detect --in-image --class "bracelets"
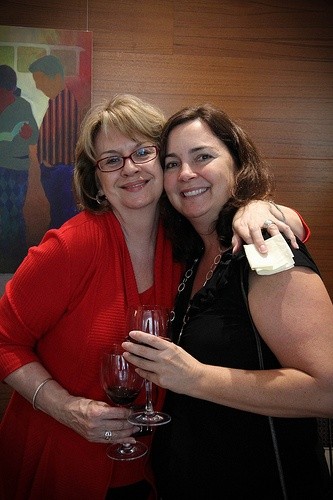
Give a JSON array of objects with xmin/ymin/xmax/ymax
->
[{"xmin": 33, "ymin": 378, "xmax": 56, "ymax": 414}]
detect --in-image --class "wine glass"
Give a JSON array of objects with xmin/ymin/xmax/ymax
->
[
  {"xmin": 127, "ymin": 305, "xmax": 171, "ymax": 426},
  {"xmin": 99, "ymin": 345, "xmax": 149, "ymax": 461}
]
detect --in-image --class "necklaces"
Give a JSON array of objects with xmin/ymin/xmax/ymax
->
[{"xmin": 169, "ymin": 241, "xmax": 233, "ymax": 347}]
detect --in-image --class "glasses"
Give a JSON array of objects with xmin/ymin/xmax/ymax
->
[{"xmin": 96, "ymin": 146, "xmax": 160, "ymax": 173}]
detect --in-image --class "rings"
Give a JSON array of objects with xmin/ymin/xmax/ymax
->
[
  {"xmin": 263, "ymin": 221, "xmax": 274, "ymax": 229},
  {"xmin": 105, "ymin": 430, "xmax": 112, "ymax": 441}
]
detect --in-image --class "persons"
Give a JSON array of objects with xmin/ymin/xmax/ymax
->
[
  {"xmin": 0, "ymin": 93, "xmax": 312, "ymax": 500},
  {"xmin": 118, "ymin": 106, "xmax": 332, "ymax": 500}
]
